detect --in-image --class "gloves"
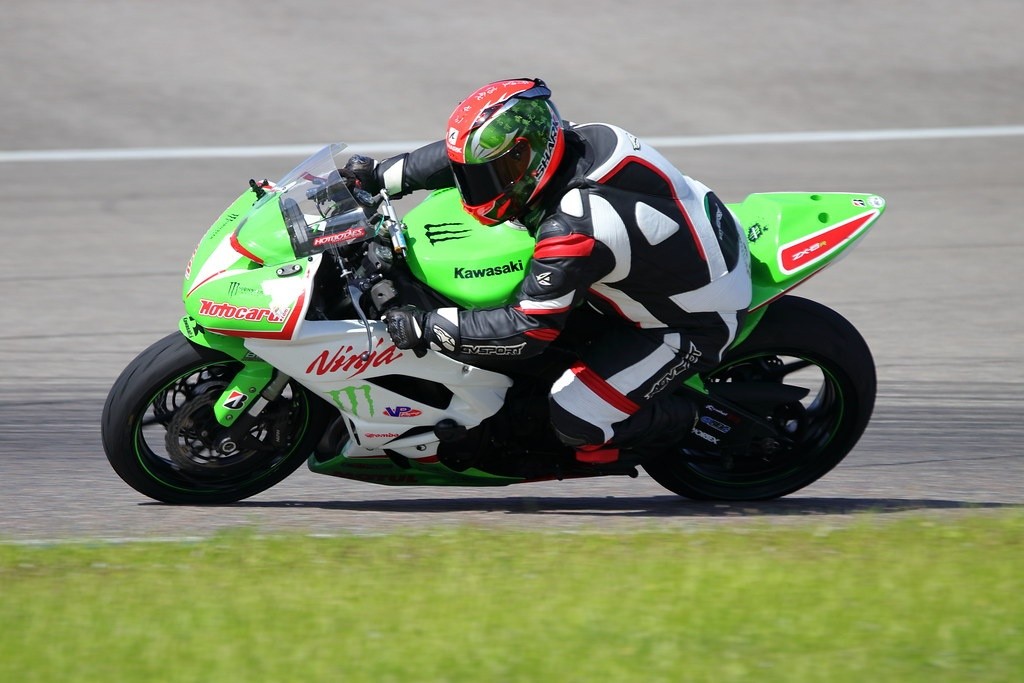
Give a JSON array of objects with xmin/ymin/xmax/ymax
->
[
  {"xmin": 381, "ymin": 304, "xmax": 462, "ymax": 355},
  {"xmin": 344, "ymin": 151, "xmax": 406, "ymax": 201}
]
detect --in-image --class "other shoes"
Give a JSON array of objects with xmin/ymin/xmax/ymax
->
[{"xmin": 637, "ymin": 395, "xmax": 701, "ymax": 451}]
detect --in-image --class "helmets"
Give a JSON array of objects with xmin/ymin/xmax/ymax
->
[{"xmin": 445, "ymin": 78, "xmax": 573, "ymax": 227}]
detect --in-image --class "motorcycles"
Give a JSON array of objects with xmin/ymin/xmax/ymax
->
[{"xmin": 99, "ymin": 142, "xmax": 886, "ymax": 507}]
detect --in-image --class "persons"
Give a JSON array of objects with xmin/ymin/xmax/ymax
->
[{"xmin": 346, "ymin": 78, "xmax": 753, "ymax": 475}]
